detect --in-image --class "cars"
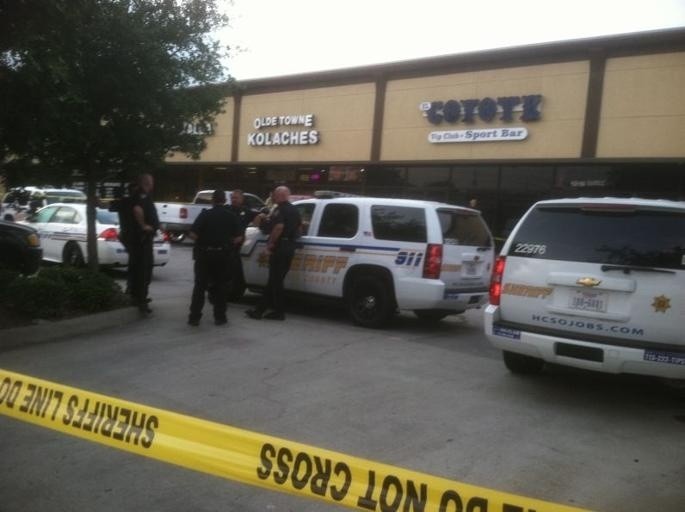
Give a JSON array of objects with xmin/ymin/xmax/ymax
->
[{"xmin": 483, "ymin": 197, "xmax": 685, "ymax": 380}]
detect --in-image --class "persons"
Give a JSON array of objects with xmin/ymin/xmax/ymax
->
[
  {"xmin": 94, "ymin": 175, "xmax": 161, "ymax": 313},
  {"xmin": 189, "ymin": 186, "xmax": 303, "ymax": 325}
]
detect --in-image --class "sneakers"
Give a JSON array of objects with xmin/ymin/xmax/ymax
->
[{"xmin": 244, "ymin": 308, "xmax": 285, "ymax": 319}]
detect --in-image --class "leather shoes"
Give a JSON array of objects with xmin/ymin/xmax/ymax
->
[
  {"xmin": 214, "ymin": 316, "xmax": 228, "ymax": 325},
  {"xmin": 187, "ymin": 320, "xmax": 200, "ymax": 326}
]
